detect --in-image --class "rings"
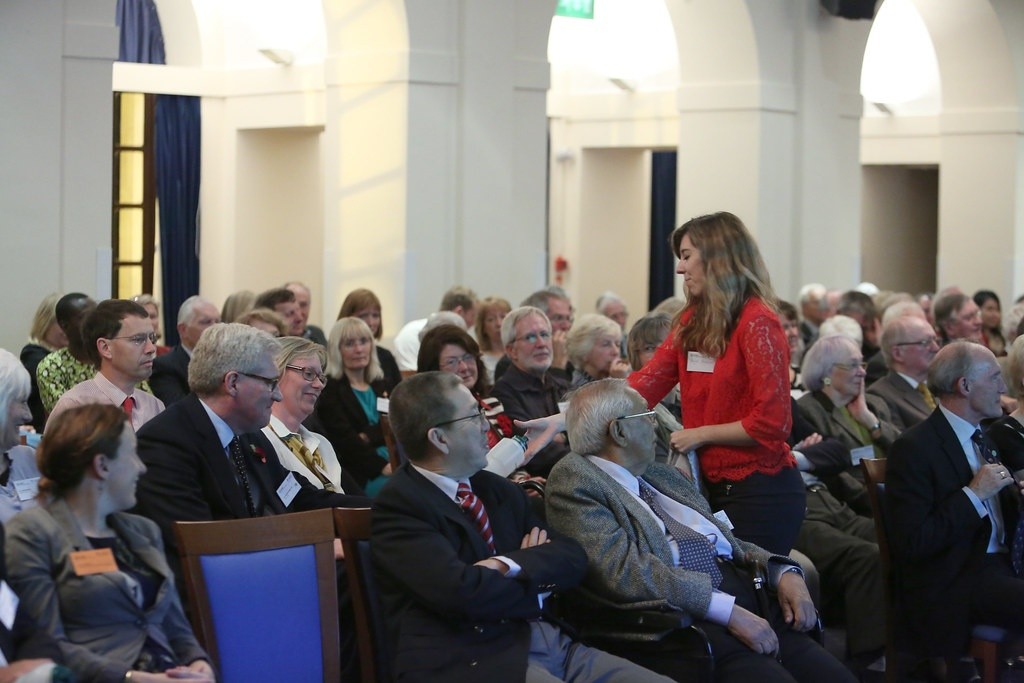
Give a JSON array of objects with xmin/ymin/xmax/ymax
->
[{"xmin": 999, "ymin": 472, "xmax": 1007, "ymax": 479}]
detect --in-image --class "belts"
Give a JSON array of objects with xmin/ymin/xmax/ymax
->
[
  {"xmin": 714, "ymin": 555, "xmax": 729, "ymax": 567},
  {"xmin": 806, "ymin": 485, "xmax": 825, "ymax": 495}
]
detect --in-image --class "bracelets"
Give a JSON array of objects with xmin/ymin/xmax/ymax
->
[
  {"xmin": 124, "ymin": 670, "xmax": 133, "ymax": 682},
  {"xmin": 788, "ymin": 568, "xmax": 799, "ymax": 572},
  {"xmin": 867, "ymin": 424, "xmax": 879, "ymax": 432}
]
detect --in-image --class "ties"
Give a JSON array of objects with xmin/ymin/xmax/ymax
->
[
  {"xmin": 228, "ymin": 435, "xmax": 258, "ymax": 519},
  {"xmin": 970, "ymin": 429, "xmax": 1024, "ymax": 576},
  {"xmin": 918, "ymin": 382, "xmax": 936, "ymax": 412},
  {"xmin": 639, "ymin": 477, "xmax": 723, "ymax": 589},
  {"xmin": 457, "ymin": 482, "xmax": 496, "ymax": 558},
  {"xmin": 122, "ymin": 396, "xmax": 134, "ymax": 433}
]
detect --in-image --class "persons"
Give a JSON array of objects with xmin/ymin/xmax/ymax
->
[
  {"xmin": 513, "ymin": 212, "xmax": 807, "ymax": 554},
  {"xmin": 0, "ymin": 282, "xmax": 1024, "ymax": 683}
]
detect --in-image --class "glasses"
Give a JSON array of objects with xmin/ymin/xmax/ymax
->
[
  {"xmin": 239, "ymin": 371, "xmax": 279, "ymax": 393},
  {"xmin": 440, "ymin": 352, "xmax": 475, "ymax": 371},
  {"xmin": 835, "ymin": 362, "xmax": 869, "ymax": 372},
  {"xmin": 432, "ymin": 407, "xmax": 486, "ymax": 429},
  {"xmin": 286, "ymin": 363, "xmax": 328, "ymax": 389},
  {"xmin": 893, "ymin": 336, "xmax": 944, "ymax": 351},
  {"xmin": 108, "ymin": 332, "xmax": 158, "ymax": 347},
  {"xmin": 511, "ymin": 330, "xmax": 551, "ymax": 343},
  {"xmin": 615, "ymin": 408, "xmax": 656, "ymax": 425},
  {"xmin": 549, "ymin": 313, "xmax": 575, "ymax": 324}
]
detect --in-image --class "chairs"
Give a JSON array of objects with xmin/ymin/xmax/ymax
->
[
  {"xmin": 334, "ymin": 507, "xmax": 381, "ymax": 683},
  {"xmin": 172, "ymin": 507, "xmax": 341, "ymax": 683},
  {"xmin": 860, "ymin": 457, "xmax": 1011, "ymax": 683}
]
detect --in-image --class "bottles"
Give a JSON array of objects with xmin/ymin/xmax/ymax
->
[{"xmin": 481, "ymin": 435, "xmax": 528, "ymax": 478}]
